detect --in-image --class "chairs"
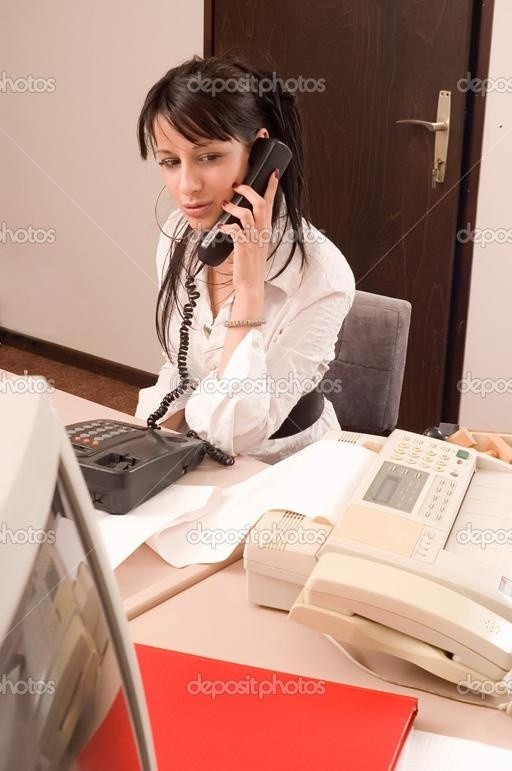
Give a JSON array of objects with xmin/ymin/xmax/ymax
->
[{"xmin": 314, "ymin": 239, "xmax": 414, "ymax": 438}]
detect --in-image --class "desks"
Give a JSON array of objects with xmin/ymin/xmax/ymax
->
[{"xmin": 2, "ymin": 368, "xmax": 512, "ymax": 771}]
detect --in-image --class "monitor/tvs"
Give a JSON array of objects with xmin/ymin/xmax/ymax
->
[{"xmin": 0, "ymin": 365, "xmax": 158, "ymax": 771}]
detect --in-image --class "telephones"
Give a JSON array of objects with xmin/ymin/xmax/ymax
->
[
  {"xmin": 197, "ymin": 138, "xmax": 293, "ymax": 266},
  {"xmin": 243, "ymin": 428, "xmax": 512, "ymax": 697}
]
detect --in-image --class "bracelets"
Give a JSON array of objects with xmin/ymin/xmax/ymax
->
[{"xmin": 222, "ymin": 318, "xmax": 270, "ymax": 329}]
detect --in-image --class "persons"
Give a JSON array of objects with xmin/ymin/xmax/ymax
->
[{"xmin": 133, "ymin": 48, "xmax": 356, "ymax": 467}]
